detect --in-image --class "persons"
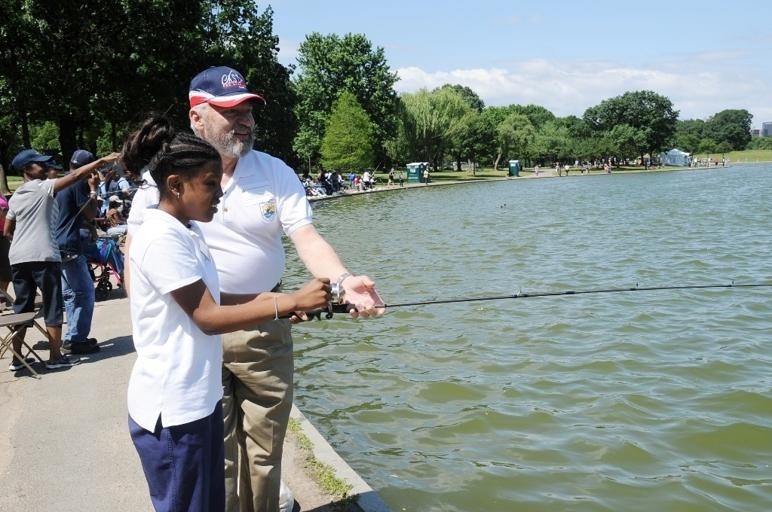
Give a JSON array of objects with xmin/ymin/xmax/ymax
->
[
  {"xmin": 552, "ymin": 157, "xmax": 612, "ymax": 176},
  {"xmin": 423, "ymin": 168, "xmax": 429, "ymax": 184},
  {"xmin": 118, "ymin": 114, "xmax": 333, "ymax": 511},
  {"xmin": 0, "ymin": 147, "xmax": 143, "ymax": 372},
  {"xmin": 123, "ymin": 63, "xmax": 386, "ymax": 511},
  {"xmin": 682, "ymin": 155, "xmax": 717, "ymax": 169},
  {"xmin": 304, "ymin": 167, "xmax": 404, "ymax": 197}
]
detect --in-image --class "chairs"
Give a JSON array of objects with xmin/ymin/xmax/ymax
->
[{"xmin": 0, "ymin": 287, "xmax": 49, "ymax": 380}]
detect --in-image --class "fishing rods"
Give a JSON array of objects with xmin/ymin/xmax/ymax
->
[
  {"xmin": 280, "ymin": 282, "xmax": 770, "ymax": 320},
  {"xmin": 68, "ymin": 96, "xmax": 183, "ymax": 225},
  {"xmin": 372, "ymin": 159, "xmax": 386, "ymax": 176}
]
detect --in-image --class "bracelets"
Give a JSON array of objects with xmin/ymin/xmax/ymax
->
[
  {"xmin": 274, "ymin": 295, "xmax": 280, "ymax": 321},
  {"xmin": 331, "ymin": 272, "xmax": 352, "ymax": 294}
]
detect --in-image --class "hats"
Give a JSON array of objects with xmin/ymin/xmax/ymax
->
[
  {"xmin": 109, "ymin": 195, "xmax": 123, "ymax": 204},
  {"xmin": 187, "ymin": 65, "xmax": 266, "ymax": 114},
  {"xmin": 97, "ymin": 196, "xmax": 105, "ymax": 202},
  {"xmin": 11, "ymin": 149, "xmax": 54, "ymax": 170},
  {"xmin": 71, "ymin": 150, "xmax": 93, "ymax": 166}
]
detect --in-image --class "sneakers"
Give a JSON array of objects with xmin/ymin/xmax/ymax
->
[
  {"xmin": 45, "ymin": 337, "xmax": 100, "ymax": 369},
  {"xmin": 8, "ymin": 358, "xmax": 35, "ymax": 370}
]
